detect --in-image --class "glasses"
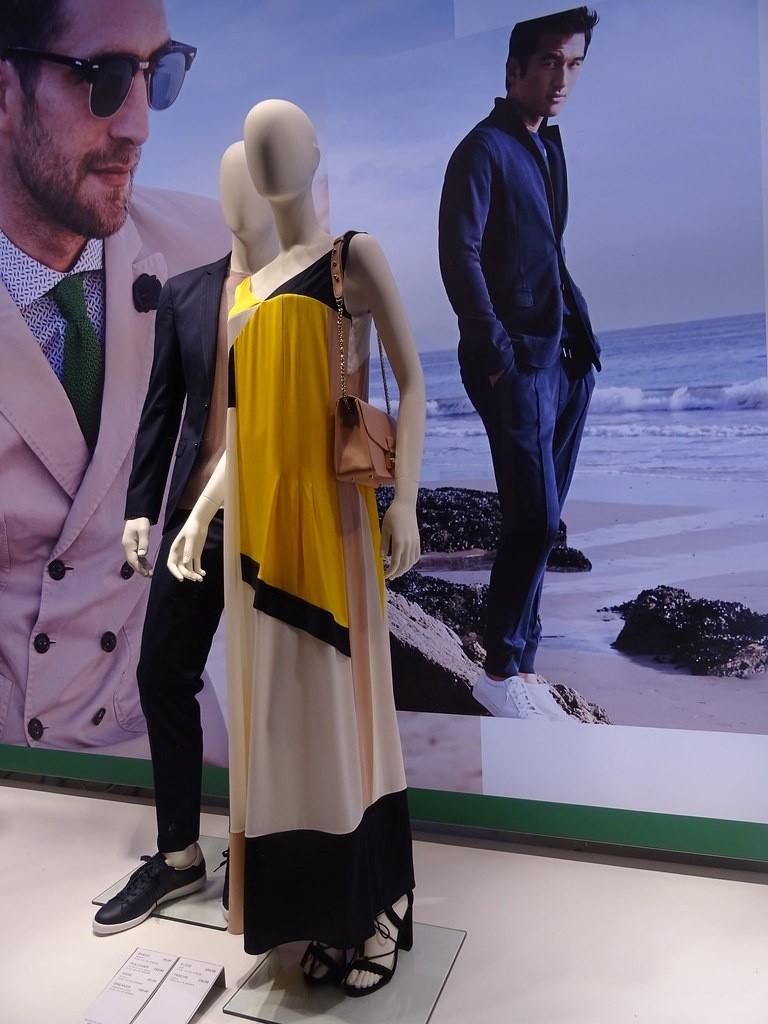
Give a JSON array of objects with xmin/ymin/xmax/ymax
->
[{"xmin": 0, "ymin": 40, "xmax": 197, "ymax": 119}]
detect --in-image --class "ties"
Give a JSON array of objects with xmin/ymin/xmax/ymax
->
[{"xmin": 44, "ymin": 272, "xmax": 105, "ymax": 458}]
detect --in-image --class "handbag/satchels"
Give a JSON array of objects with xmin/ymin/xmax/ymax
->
[{"xmin": 334, "ymin": 395, "xmax": 398, "ymax": 487}]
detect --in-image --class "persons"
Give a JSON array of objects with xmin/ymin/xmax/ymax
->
[
  {"xmin": 89, "ymin": 97, "xmax": 427, "ymax": 998},
  {"xmin": 435, "ymin": 4, "xmax": 604, "ymax": 723},
  {"xmin": 0, "ymin": 1, "xmax": 233, "ymax": 762}
]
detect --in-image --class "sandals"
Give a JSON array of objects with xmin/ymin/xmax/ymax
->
[
  {"xmin": 341, "ymin": 889, "xmax": 414, "ymax": 997},
  {"xmin": 300, "ymin": 939, "xmax": 364, "ymax": 985}
]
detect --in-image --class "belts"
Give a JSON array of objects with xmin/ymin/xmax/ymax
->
[{"xmin": 558, "ymin": 347, "xmax": 581, "ymax": 359}]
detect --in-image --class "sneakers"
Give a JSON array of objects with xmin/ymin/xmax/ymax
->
[
  {"xmin": 214, "ymin": 846, "xmax": 230, "ymax": 921},
  {"xmin": 472, "ymin": 669, "xmax": 578, "ymax": 722},
  {"xmin": 93, "ymin": 842, "xmax": 207, "ymax": 934}
]
